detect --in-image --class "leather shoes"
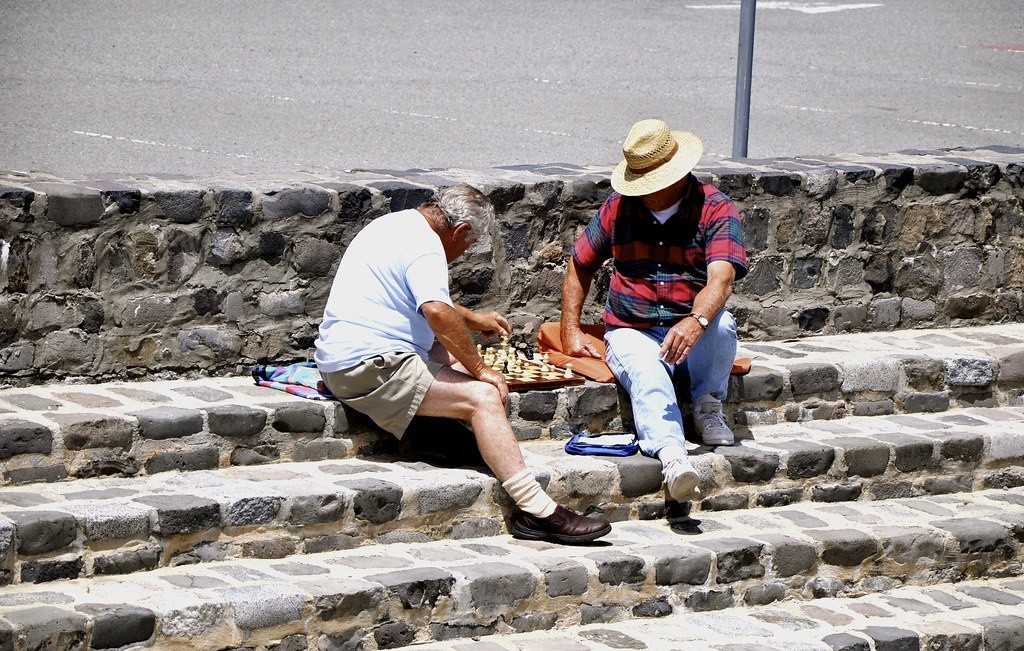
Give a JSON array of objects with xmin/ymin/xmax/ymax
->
[
  {"xmin": 441, "ymin": 418, "xmax": 480, "ymax": 460},
  {"xmin": 512, "ymin": 505, "xmax": 612, "ymax": 542}
]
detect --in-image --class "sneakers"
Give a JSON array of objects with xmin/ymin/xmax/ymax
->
[
  {"xmin": 661, "ymin": 457, "xmax": 701, "ymax": 504},
  {"xmin": 693, "ymin": 403, "xmax": 734, "ymax": 445}
]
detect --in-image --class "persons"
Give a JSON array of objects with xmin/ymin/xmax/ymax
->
[
  {"xmin": 315, "ymin": 184, "xmax": 613, "ymax": 543},
  {"xmin": 561, "ymin": 118, "xmax": 749, "ymax": 503}
]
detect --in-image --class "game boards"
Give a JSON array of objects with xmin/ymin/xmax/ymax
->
[{"xmin": 462, "ymin": 345, "xmax": 588, "ymax": 393}]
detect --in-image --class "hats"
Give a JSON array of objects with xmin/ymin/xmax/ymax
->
[{"xmin": 611, "ymin": 119, "xmax": 702, "ymax": 197}]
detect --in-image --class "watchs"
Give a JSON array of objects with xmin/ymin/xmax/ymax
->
[{"xmin": 688, "ymin": 313, "xmax": 709, "ymax": 331}]
